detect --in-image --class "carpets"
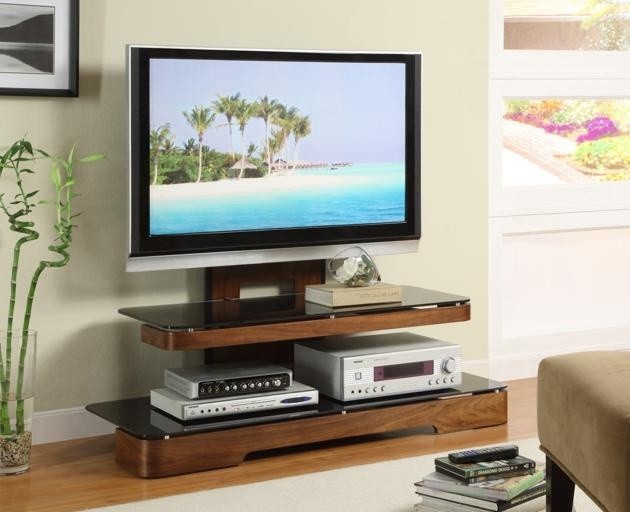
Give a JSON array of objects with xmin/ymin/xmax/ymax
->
[{"xmin": 78, "ymin": 437, "xmax": 603, "ymax": 512}]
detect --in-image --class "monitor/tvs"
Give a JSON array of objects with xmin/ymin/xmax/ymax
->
[{"xmin": 126, "ymin": 43, "xmax": 423, "ymax": 273}]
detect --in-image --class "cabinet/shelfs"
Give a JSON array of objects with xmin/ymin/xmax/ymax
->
[{"xmin": 86, "ymin": 281, "xmax": 507, "ymax": 480}]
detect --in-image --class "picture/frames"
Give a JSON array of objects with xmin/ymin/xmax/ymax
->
[{"xmin": 0, "ymin": 0, "xmax": 79, "ymax": 97}]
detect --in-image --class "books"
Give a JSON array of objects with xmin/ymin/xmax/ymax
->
[
  {"xmin": 305, "ymin": 281, "xmax": 401, "ymax": 308},
  {"xmin": 413, "ymin": 454, "xmax": 547, "ymax": 512}
]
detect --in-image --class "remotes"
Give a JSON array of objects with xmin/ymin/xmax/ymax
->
[{"xmin": 453, "ymin": 445, "xmax": 518, "ymax": 464}]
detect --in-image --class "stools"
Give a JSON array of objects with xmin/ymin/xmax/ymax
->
[{"xmin": 536, "ymin": 349, "xmax": 630, "ymax": 512}]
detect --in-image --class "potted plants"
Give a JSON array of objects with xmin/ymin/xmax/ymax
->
[{"xmin": 0, "ymin": 134, "xmax": 107, "ymax": 477}]
